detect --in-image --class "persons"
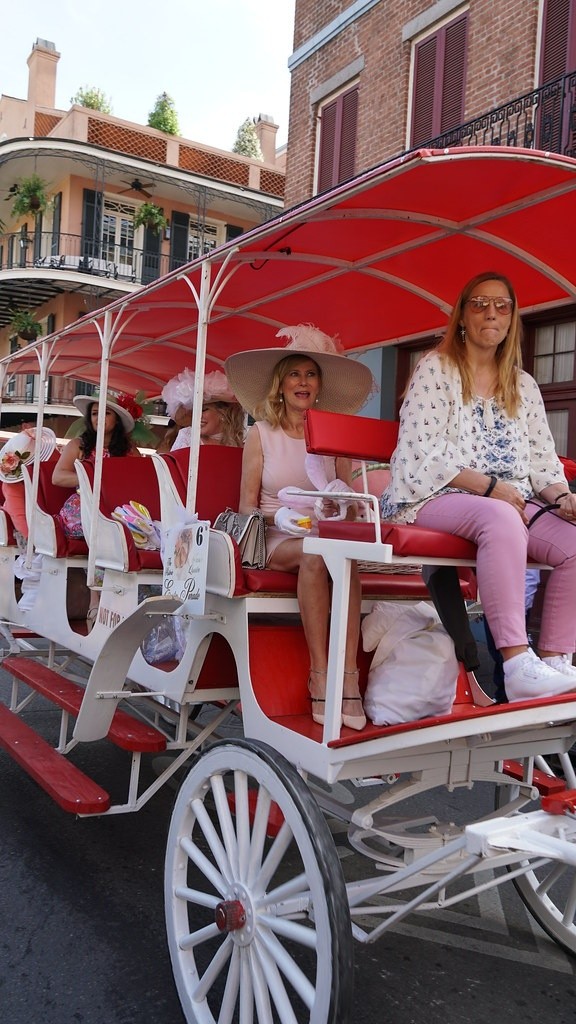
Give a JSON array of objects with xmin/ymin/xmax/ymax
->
[
  {"xmin": 51, "ymin": 393, "xmax": 141, "ymax": 631},
  {"xmin": 162, "ymin": 369, "xmax": 245, "ymax": 452},
  {"xmin": 382, "ymin": 271, "xmax": 576, "ymax": 703},
  {"xmin": 0, "ymin": 427, "xmax": 56, "ymax": 549},
  {"xmin": 225, "ymin": 326, "xmax": 373, "ymax": 730}
]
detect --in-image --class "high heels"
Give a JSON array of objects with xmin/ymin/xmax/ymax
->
[
  {"xmin": 86, "ymin": 607, "xmax": 98, "ymax": 634},
  {"xmin": 341, "ymin": 669, "xmax": 367, "ymax": 730},
  {"xmin": 307, "ymin": 669, "xmax": 327, "ymax": 725}
]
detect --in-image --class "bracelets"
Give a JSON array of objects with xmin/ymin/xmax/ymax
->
[
  {"xmin": 554, "ymin": 493, "xmax": 568, "ymax": 503},
  {"xmin": 483, "ymin": 476, "xmax": 497, "ymax": 496}
]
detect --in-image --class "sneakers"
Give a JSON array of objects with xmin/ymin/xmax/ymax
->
[
  {"xmin": 502, "ymin": 647, "xmax": 576, "ymax": 704},
  {"xmin": 540, "ymin": 654, "xmax": 576, "ymax": 677}
]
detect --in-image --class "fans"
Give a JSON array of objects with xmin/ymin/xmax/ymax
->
[{"xmin": 117, "ymin": 178, "xmax": 156, "ymax": 198}]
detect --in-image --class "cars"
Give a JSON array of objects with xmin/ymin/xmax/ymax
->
[{"xmin": 0, "ymin": 403, "xmax": 171, "ymax": 456}]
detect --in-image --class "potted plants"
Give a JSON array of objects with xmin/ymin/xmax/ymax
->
[
  {"xmin": 11, "ymin": 176, "xmax": 55, "ymax": 223},
  {"xmin": 7, "ymin": 307, "xmax": 43, "ymax": 341},
  {"xmin": 132, "ymin": 203, "xmax": 166, "ymax": 233}
]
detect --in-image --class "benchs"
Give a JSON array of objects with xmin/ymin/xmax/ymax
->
[
  {"xmin": 72, "ymin": 458, "xmax": 164, "ymax": 636},
  {"xmin": 303, "ymin": 408, "xmax": 576, "ymax": 743},
  {"xmin": 150, "ymin": 444, "xmax": 333, "ymax": 719},
  {"xmin": 1, "ymin": 436, "xmax": 91, "ymax": 625}
]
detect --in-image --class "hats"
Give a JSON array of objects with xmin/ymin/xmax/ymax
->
[
  {"xmin": 160, "ymin": 367, "xmax": 239, "ymax": 427},
  {"xmin": 0, "ymin": 427, "xmax": 56, "ymax": 484},
  {"xmin": 223, "ymin": 322, "xmax": 379, "ymax": 424},
  {"xmin": 64, "ymin": 390, "xmax": 160, "ymax": 446}
]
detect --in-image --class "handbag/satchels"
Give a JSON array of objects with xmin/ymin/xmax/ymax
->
[
  {"xmin": 60, "ymin": 493, "xmax": 84, "ymax": 539},
  {"xmin": 212, "ymin": 507, "xmax": 265, "ymax": 570}
]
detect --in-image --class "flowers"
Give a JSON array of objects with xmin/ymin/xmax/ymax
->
[
  {"xmin": 115, "ymin": 391, "xmax": 157, "ymax": 444},
  {"xmin": 0, "ymin": 450, "xmax": 31, "ymax": 478}
]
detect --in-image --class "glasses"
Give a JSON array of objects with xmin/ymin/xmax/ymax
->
[{"xmin": 463, "ymin": 296, "xmax": 514, "ymax": 315}]
